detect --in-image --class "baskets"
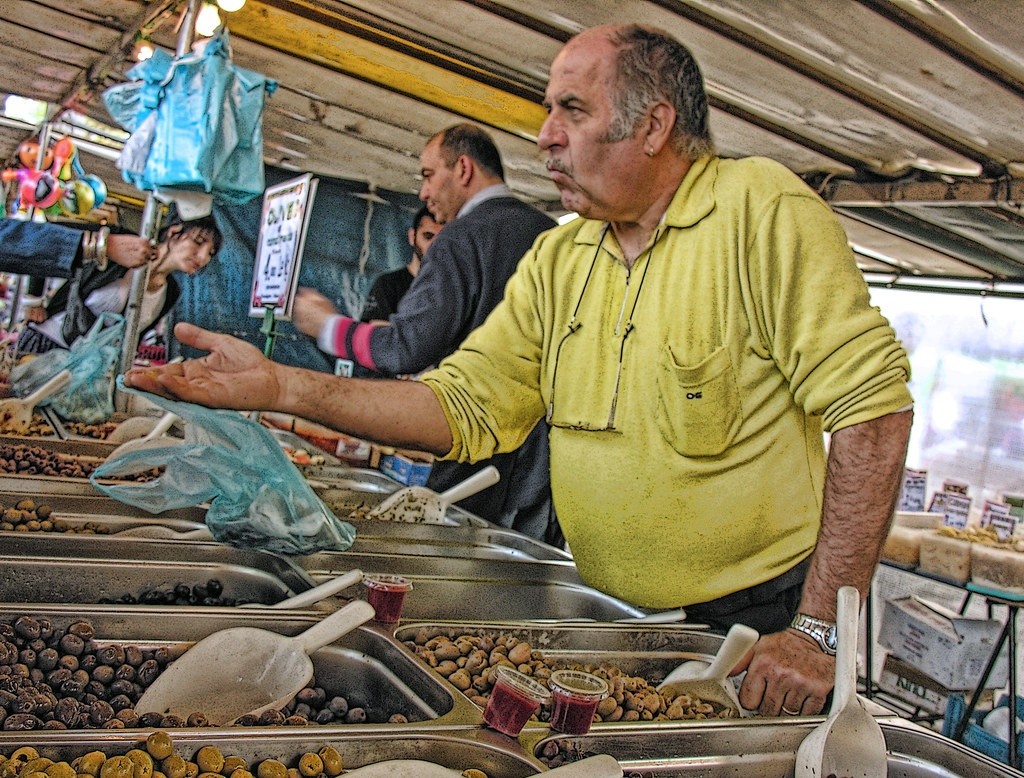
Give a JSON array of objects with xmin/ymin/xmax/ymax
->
[{"xmin": 942, "ymin": 694, "xmax": 1024, "ymax": 769}]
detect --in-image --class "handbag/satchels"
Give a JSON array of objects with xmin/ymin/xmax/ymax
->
[
  {"xmin": 89, "ymin": 372, "xmax": 358, "ymax": 556},
  {"xmin": 63, "ymin": 267, "xmax": 107, "ymax": 344},
  {"xmin": 11, "ymin": 311, "xmax": 126, "ymax": 424}
]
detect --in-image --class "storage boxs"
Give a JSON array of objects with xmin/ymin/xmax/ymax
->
[
  {"xmin": 964, "ymin": 709, "xmax": 1024, "ymax": 774},
  {"xmin": 881, "ymin": 653, "xmax": 993, "ymax": 715},
  {"xmin": 878, "ymin": 594, "xmax": 1010, "ymax": 692},
  {"xmin": 882, "ymin": 521, "xmax": 1024, "ymax": 601},
  {"xmin": 260, "ymin": 413, "xmax": 435, "ymax": 486}
]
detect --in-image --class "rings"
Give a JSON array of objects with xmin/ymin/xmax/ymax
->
[{"xmin": 782, "ymin": 706, "xmax": 799, "ymax": 716}]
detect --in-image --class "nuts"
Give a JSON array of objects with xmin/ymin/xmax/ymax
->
[{"xmin": 0, "ymin": 419, "xmax": 738, "ymax": 778}]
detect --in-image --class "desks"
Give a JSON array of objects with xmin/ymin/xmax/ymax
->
[{"xmin": 866, "ymin": 556, "xmax": 1024, "ymax": 768}]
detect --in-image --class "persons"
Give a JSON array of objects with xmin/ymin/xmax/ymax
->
[
  {"xmin": 122, "ymin": 21, "xmax": 915, "ymax": 722},
  {"xmin": 16, "ymin": 210, "xmax": 223, "ymax": 360},
  {"xmin": 292, "ymin": 124, "xmax": 559, "ymax": 551},
  {"xmin": 348, "ymin": 206, "xmax": 448, "ymax": 379},
  {"xmin": 0, "ymin": 218, "xmax": 158, "ymax": 279}
]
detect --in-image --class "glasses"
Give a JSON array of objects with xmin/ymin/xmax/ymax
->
[{"xmin": 544, "ymin": 325, "xmax": 637, "ymax": 434}]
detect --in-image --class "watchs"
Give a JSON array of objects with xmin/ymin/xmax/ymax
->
[{"xmin": 791, "ymin": 613, "xmax": 838, "ymax": 656}]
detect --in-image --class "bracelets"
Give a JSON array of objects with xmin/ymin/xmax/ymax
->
[{"xmin": 83, "ymin": 226, "xmax": 110, "ymax": 271}]
